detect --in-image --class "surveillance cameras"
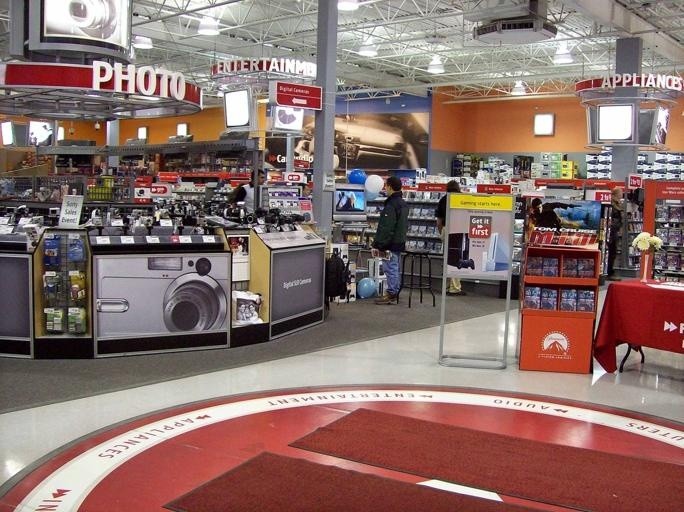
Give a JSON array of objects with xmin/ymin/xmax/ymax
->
[
  {"xmin": 68, "ymin": 127, "xmax": 74, "ymax": 135},
  {"xmin": 94, "ymin": 122, "xmax": 101, "ymax": 131}
]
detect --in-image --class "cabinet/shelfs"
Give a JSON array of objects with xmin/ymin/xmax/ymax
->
[
  {"xmin": 515, "ymin": 198, "xmax": 602, "ymax": 374},
  {"xmin": 0, "ymin": 137, "xmax": 684, "ymax": 310}
]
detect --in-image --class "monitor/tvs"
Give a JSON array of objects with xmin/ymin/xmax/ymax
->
[
  {"xmin": 1, "ymin": 121, "xmax": 15, "ymax": 146},
  {"xmin": 176, "ymin": 123, "xmax": 187, "ymax": 136},
  {"xmin": 137, "ymin": 127, "xmax": 147, "ymax": 140},
  {"xmin": 272, "ymin": 106, "xmax": 304, "ymax": 131},
  {"xmin": 27, "ymin": 0, "xmax": 134, "ymax": 64},
  {"xmin": 223, "ymin": 89, "xmax": 255, "ymax": 132},
  {"xmin": 332, "ymin": 189, "xmax": 367, "ymax": 214},
  {"xmin": 585, "ymin": 102, "xmax": 672, "ymax": 144},
  {"xmin": 25, "ymin": 120, "xmax": 57, "ymax": 148},
  {"xmin": 532, "ymin": 113, "xmax": 554, "ymax": 135}
]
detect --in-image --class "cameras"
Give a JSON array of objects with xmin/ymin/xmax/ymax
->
[
  {"xmin": 95, "ymin": 257, "xmax": 231, "ymax": 342},
  {"xmin": 44, "ymin": 0, "xmax": 129, "ymax": 48}
]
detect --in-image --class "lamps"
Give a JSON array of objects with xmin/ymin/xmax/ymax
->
[
  {"xmin": 197, "ymin": 3, "xmax": 220, "ymax": 37},
  {"xmin": 286, "ymin": 58, "xmax": 318, "ymax": 78},
  {"xmin": 338, "ymin": 2, "xmax": 363, "ymax": 12},
  {"xmin": 553, "ymin": 41, "xmax": 574, "ymax": 65},
  {"xmin": 133, "ymin": 35, "xmax": 154, "ymax": 50},
  {"xmin": 427, "ymin": 54, "xmax": 445, "ymax": 75},
  {"xmin": 511, "ymin": 81, "xmax": 527, "ymax": 97},
  {"xmin": 358, "ymin": 35, "xmax": 378, "ymax": 58}
]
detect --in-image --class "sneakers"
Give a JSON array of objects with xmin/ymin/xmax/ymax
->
[
  {"xmin": 446, "ymin": 290, "xmax": 468, "ymax": 296},
  {"xmin": 375, "ymin": 291, "xmax": 392, "ymax": 298},
  {"xmin": 375, "ymin": 295, "xmax": 398, "ymax": 305}
]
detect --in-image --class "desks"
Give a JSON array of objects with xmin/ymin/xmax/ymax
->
[{"xmin": 591, "ymin": 276, "xmax": 684, "ymax": 372}]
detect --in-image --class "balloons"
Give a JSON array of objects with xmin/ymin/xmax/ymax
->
[
  {"xmin": 357, "ymin": 277, "xmax": 375, "ymax": 299},
  {"xmin": 347, "ymin": 169, "xmax": 384, "ymax": 200}
]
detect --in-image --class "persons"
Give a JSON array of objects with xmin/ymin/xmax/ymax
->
[
  {"xmin": 529, "ymin": 198, "xmax": 582, "ymax": 230},
  {"xmin": 435, "ymin": 181, "xmax": 466, "ymax": 295},
  {"xmin": 340, "ymin": 192, "xmax": 355, "ymax": 210},
  {"xmin": 605, "ymin": 188, "xmax": 624, "ymax": 281},
  {"xmin": 371, "ymin": 177, "xmax": 409, "ymax": 305},
  {"xmin": 237, "ymin": 303, "xmax": 258, "ymax": 321},
  {"xmin": 235, "ymin": 170, "xmax": 265, "ymax": 213}
]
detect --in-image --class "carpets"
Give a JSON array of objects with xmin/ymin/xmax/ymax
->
[
  {"xmin": 159, "ymin": 448, "xmax": 550, "ymax": 512},
  {"xmin": 285, "ymin": 406, "xmax": 684, "ymax": 511}
]
